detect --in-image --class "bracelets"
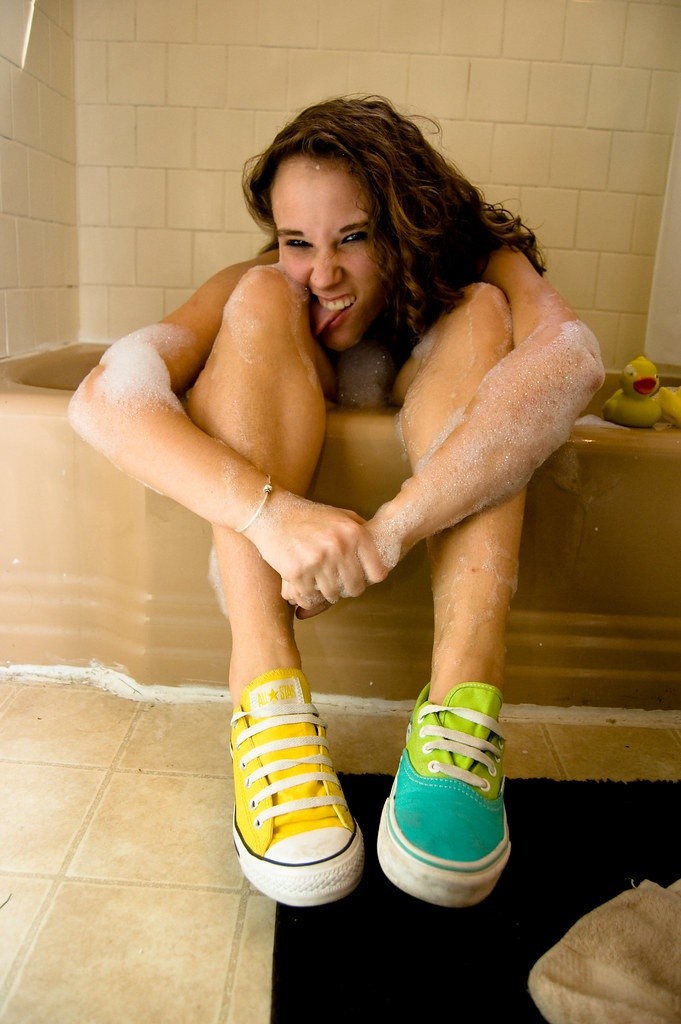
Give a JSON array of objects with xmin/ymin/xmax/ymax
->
[{"xmin": 237, "ymin": 476, "xmax": 273, "ymax": 537}]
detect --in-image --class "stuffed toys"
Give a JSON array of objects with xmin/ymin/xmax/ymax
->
[{"xmin": 600, "ymin": 356, "xmax": 681, "ymax": 432}]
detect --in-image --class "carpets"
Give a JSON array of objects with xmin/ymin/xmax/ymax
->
[{"xmin": 269, "ymin": 776, "xmax": 681, "ymax": 1024}]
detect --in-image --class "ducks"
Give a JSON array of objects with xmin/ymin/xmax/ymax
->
[{"xmin": 603, "ymin": 356, "xmax": 662, "ymax": 430}]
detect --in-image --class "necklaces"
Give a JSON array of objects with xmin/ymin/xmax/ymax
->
[{"xmin": 336, "ymin": 356, "xmax": 387, "ymax": 408}]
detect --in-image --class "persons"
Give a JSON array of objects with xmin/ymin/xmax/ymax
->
[{"xmin": 67, "ymin": 95, "xmax": 604, "ymax": 907}]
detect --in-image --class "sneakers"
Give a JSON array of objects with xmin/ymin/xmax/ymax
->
[{"xmin": 230, "ymin": 670, "xmax": 513, "ymax": 908}]
